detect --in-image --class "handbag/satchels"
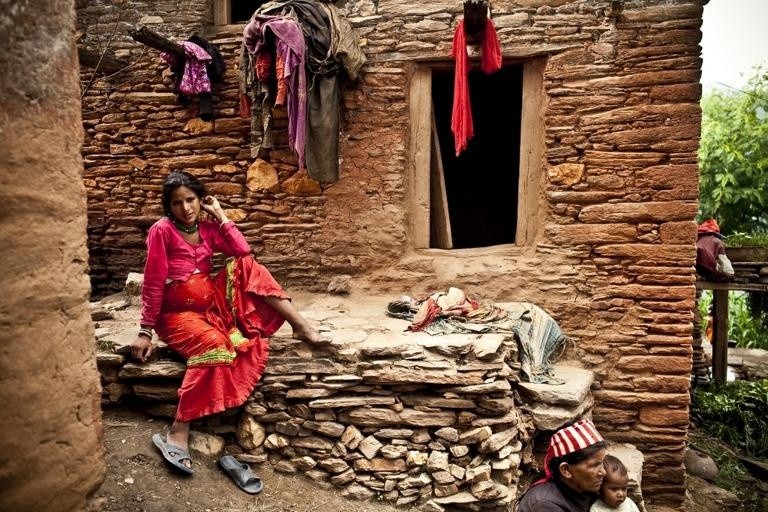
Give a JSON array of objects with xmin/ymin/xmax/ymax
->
[{"xmin": 715, "ymin": 254, "xmax": 735, "ymax": 278}]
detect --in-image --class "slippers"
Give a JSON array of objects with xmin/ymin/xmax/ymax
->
[
  {"xmin": 152, "ymin": 434, "xmax": 194, "ymax": 474},
  {"xmin": 219, "ymin": 455, "xmax": 264, "ymax": 494}
]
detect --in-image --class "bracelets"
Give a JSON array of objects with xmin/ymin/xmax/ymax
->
[
  {"xmin": 220, "ymin": 220, "xmax": 232, "ymax": 228},
  {"xmin": 138, "ymin": 329, "xmax": 153, "ymax": 341}
]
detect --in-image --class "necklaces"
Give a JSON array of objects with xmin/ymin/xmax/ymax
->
[{"xmin": 172, "ymin": 219, "xmax": 198, "ymax": 233}]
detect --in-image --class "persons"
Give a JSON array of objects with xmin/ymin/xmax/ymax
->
[
  {"xmin": 516, "ymin": 416, "xmax": 608, "ymax": 512},
  {"xmin": 132, "ymin": 172, "xmax": 332, "ymax": 469},
  {"xmin": 589, "ymin": 455, "xmax": 640, "ymax": 512}
]
